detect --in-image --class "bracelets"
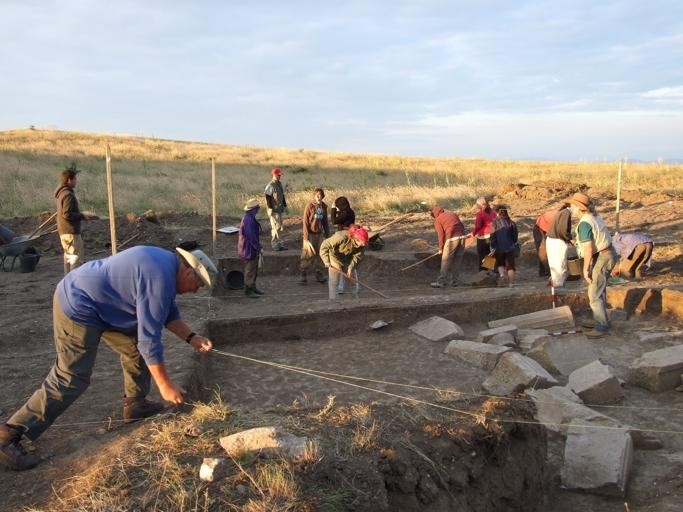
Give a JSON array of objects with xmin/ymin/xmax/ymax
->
[{"xmin": 185, "ymin": 332, "xmax": 195, "ymax": 344}]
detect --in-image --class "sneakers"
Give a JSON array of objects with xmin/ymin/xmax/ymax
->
[
  {"xmin": 584, "ymin": 331, "xmax": 609, "ymax": 339},
  {"xmin": 0, "ymin": 424, "xmax": 40, "ymax": 469},
  {"xmin": 123, "ymin": 394, "xmax": 164, "ymax": 423},
  {"xmin": 582, "ymin": 319, "xmax": 596, "ymax": 327},
  {"xmin": 430, "ymin": 282, "xmax": 448, "ymax": 288}
]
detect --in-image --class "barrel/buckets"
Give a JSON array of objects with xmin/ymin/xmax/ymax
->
[
  {"xmin": 226, "ymin": 270, "xmax": 244, "ymax": 289},
  {"xmin": 19, "ymin": 253, "xmax": 40, "ymax": 272},
  {"xmin": 568, "ymin": 255, "xmax": 582, "ymax": 277}
]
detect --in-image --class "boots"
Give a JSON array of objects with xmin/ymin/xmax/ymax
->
[{"xmin": 244, "ymin": 286, "xmax": 264, "ymax": 298}]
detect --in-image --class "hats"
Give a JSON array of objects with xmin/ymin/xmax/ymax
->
[
  {"xmin": 563, "ymin": 193, "xmax": 589, "ymax": 211},
  {"xmin": 271, "ymin": 168, "xmax": 284, "ymax": 176},
  {"xmin": 175, "ymin": 247, "xmax": 217, "ymax": 289},
  {"xmin": 244, "ymin": 199, "xmax": 260, "ymax": 211}
]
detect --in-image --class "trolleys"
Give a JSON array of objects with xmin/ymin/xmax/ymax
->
[
  {"xmin": 363, "ymin": 212, "xmax": 416, "ymax": 250},
  {"xmin": 0, "ymin": 222, "xmax": 58, "ymax": 273}
]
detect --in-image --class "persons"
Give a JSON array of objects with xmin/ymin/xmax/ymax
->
[
  {"xmin": 318, "ymin": 224, "xmax": 369, "ymax": 309},
  {"xmin": 53, "ymin": 167, "xmax": 93, "ymax": 275},
  {"xmin": 1, "ymin": 244, "xmax": 218, "ymax": 471},
  {"xmin": 237, "ymin": 198, "xmax": 264, "ymax": 298},
  {"xmin": 263, "ymin": 167, "xmax": 290, "ymax": 251},
  {"xmin": 562, "ymin": 192, "xmax": 618, "ymax": 338},
  {"xmin": 330, "ymin": 196, "xmax": 359, "ymax": 294},
  {"xmin": 298, "ymin": 188, "xmax": 330, "ymax": 286},
  {"xmin": 430, "ymin": 195, "xmax": 656, "ymax": 289}
]
